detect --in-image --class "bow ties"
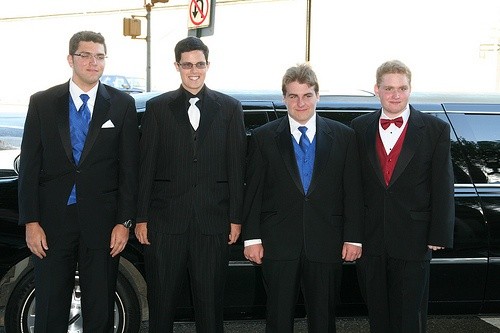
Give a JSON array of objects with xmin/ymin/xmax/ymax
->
[{"xmin": 379, "ymin": 116, "xmax": 403, "ymax": 130}]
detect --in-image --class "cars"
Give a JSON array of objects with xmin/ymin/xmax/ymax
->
[{"xmin": 0, "ymin": 91, "xmax": 500, "ymax": 333}]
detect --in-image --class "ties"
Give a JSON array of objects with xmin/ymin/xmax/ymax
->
[
  {"xmin": 187, "ymin": 97, "xmax": 201, "ymax": 130},
  {"xmin": 298, "ymin": 127, "xmax": 310, "ymax": 153},
  {"xmin": 77, "ymin": 93, "xmax": 91, "ymax": 125}
]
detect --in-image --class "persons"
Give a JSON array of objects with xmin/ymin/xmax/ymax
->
[
  {"xmin": 350, "ymin": 60, "xmax": 455, "ymax": 331},
  {"xmin": 18, "ymin": 31, "xmax": 138, "ymax": 332},
  {"xmin": 134, "ymin": 36, "xmax": 247, "ymax": 333},
  {"xmin": 241, "ymin": 65, "xmax": 364, "ymax": 333}
]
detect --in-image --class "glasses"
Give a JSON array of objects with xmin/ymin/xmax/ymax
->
[
  {"xmin": 176, "ymin": 61, "xmax": 208, "ymax": 70},
  {"xmin": 69, "ymin": 51, "xmax": 107, "ymax": 62}
]
